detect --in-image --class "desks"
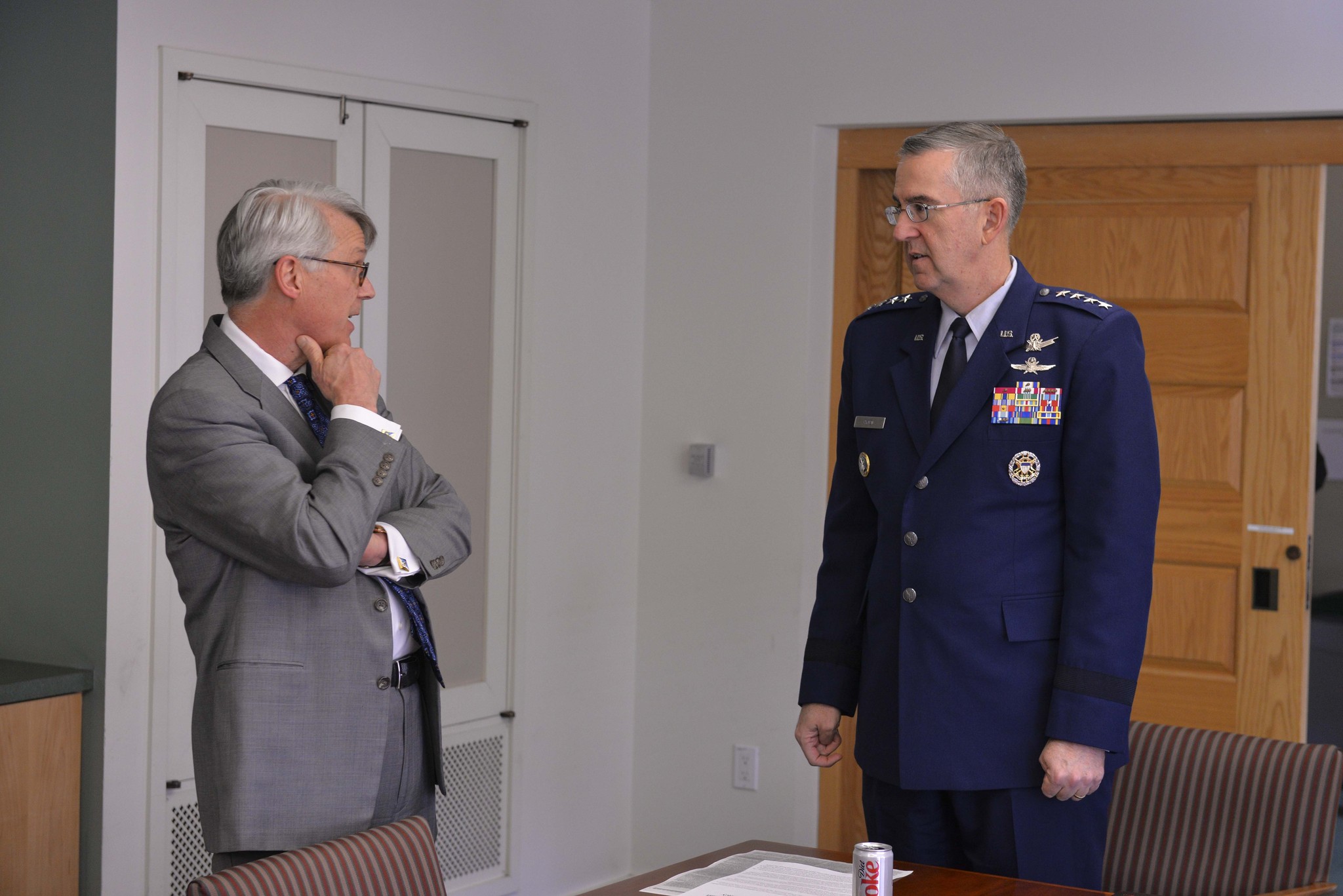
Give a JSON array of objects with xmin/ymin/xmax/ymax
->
[{"xmin": 568, "ymin": 838, "xmax": 1125, "ymax": 896}]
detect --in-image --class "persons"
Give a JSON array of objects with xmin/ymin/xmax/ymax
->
[
  {"xmin": 144, "ymin": 179, "xmax": 476, "ymax": 877},
  {"xmin": 791, "ymin": 117, "xmax": 1162, "ymax": 895}
]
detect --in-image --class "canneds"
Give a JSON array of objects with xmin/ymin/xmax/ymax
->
[{"xmin": 853, "ymin": 842, "xmax": 893, "ymax": 896}]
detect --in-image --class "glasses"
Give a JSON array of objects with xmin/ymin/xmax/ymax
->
[
  {"xmin": 273, "ymin": 255, "xmax": 370, "ymax": 287},
  {"xmin": 884, "ymin": 198, "xmax": 991, "ymax": 226}
]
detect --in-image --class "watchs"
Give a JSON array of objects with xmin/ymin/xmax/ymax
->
[{"xmin": 372, "ymin": 524, "xmax": 387, "ymax": 534}]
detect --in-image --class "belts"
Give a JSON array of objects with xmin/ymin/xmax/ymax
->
[{"xmin": 390, "ymin": 652, "xmax": 423, "ymax": 689}]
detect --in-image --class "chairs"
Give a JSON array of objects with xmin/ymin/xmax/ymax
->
[
  {"xmin": 182, "ymin": 816, "xmax": 447, "ymax": 896},
  {"xmin": 1106, "ymin": 718, "xmax": 1343, "ymax": 896}
]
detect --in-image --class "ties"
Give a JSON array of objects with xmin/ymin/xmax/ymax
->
[
  {"xmin": 283, "ymin": 373, "xmax": 446, "ymax": 690},
  {"xmin": 928, "ymin": 318, "xmax": 973, "ymax": 438}
]
